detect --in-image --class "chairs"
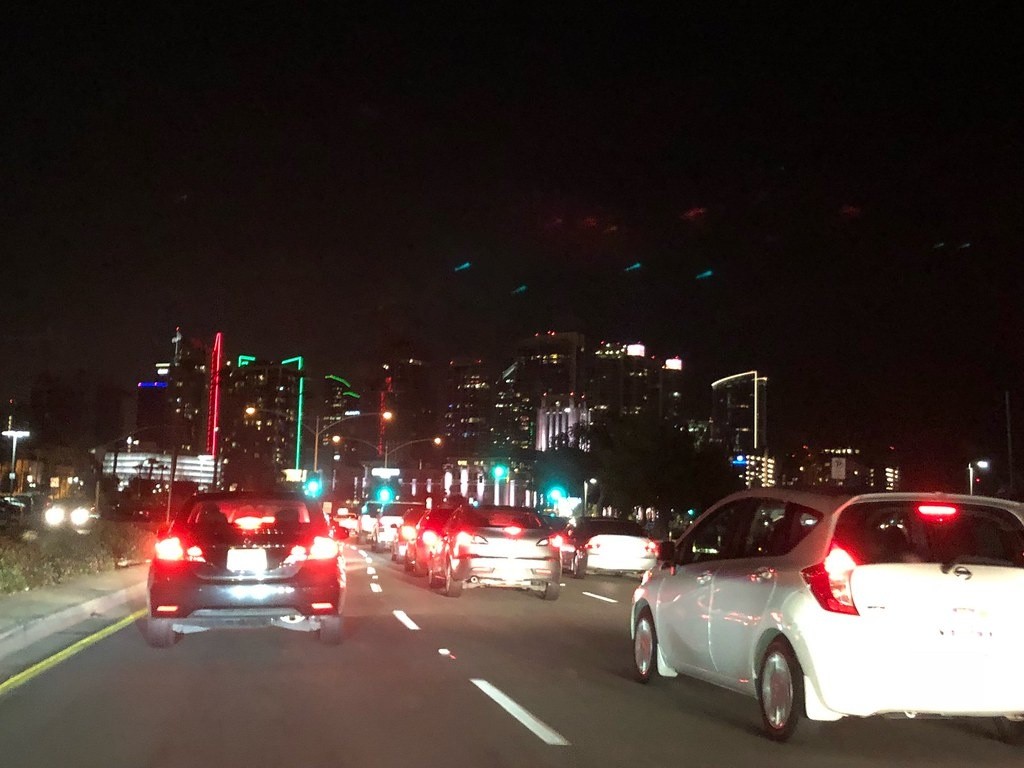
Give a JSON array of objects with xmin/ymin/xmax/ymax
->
[
  {"xmin": 199, "ymin": 511, "xmax": 226, "ymax": 523},
  {"xmin": 767, "ymin": 516, "xmax": 803, "ymax": 552},
  {"xmin": 866, "ymin": 527, "xmax": 907, "ymax": 555},
  {"xmin": 275, "ymin": 509, "xmax": 299, "ymax": 523},
  {"xmin": 963, "ymin": 518, "xmax": 1011, "ymax": 556}
]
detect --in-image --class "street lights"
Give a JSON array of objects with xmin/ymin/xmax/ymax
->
[
  {"xmin": 968, "ymin": 458, "xmax": 988, "ymax": 495},
  {"xmin": 331, "ymin": 435, "xmax": 440, "ymax": 503},
  {"xmin": 3, "ymin": 431, "xmax": 30, "ymax": 500},
  {"xmin": 245, "ymin": 405, "xmax": 392, "ymax": 502}
]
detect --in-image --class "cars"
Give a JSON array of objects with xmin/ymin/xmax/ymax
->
[
  {"xmin": 630, "ymin": 488, "xmax": 1024, "ymax": 742},
  {"xmin": 146, "ymin": 491, "xmax": 350, "ymax": 646},
  {"xmin": 334, "ymin": 501, "xmax": 587, "ymax": 601},
  {"xmin": 559, "ymin": 516, "xmax": 659, "ymax": 580},
  {"xmin": 0, "ymin": 495, "xmax": 153, "ymax": 537}
]
